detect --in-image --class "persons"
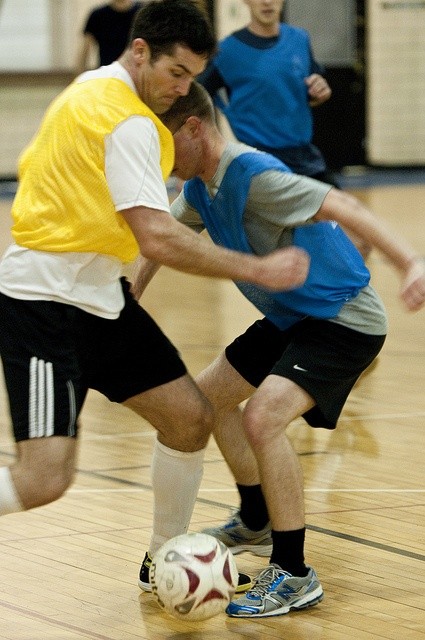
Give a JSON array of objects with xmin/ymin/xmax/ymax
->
[
  {"xmin": 155, "ymin": 80, "xmax": 425, "ymax": 621},
  {"xmin": 0, "ymin": 1, "xmax": 310, "ymax": 592},
  {"xmin": 75, "ymin": 0, "xmax": 145, "ymax": 71},
  {"xmin": 198, "ymin": 0, "xmax": 373, "ymax": 264}
]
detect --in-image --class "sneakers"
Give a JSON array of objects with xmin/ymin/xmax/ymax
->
[
  {"xmin": 137, "ymin": 550, "xmax": 251, "ymax": 594},
  {"xmin": 192, "ymin": 510, "xmax": 274, "ymax": 558},
  {"xmin": 225, "ymin": 563, "xmax": 325, "ymax": 618}
]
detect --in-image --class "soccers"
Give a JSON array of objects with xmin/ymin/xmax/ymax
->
[{"xmin": 150, "ymin": 533, "xmax": 237, "ymax": 621}]
